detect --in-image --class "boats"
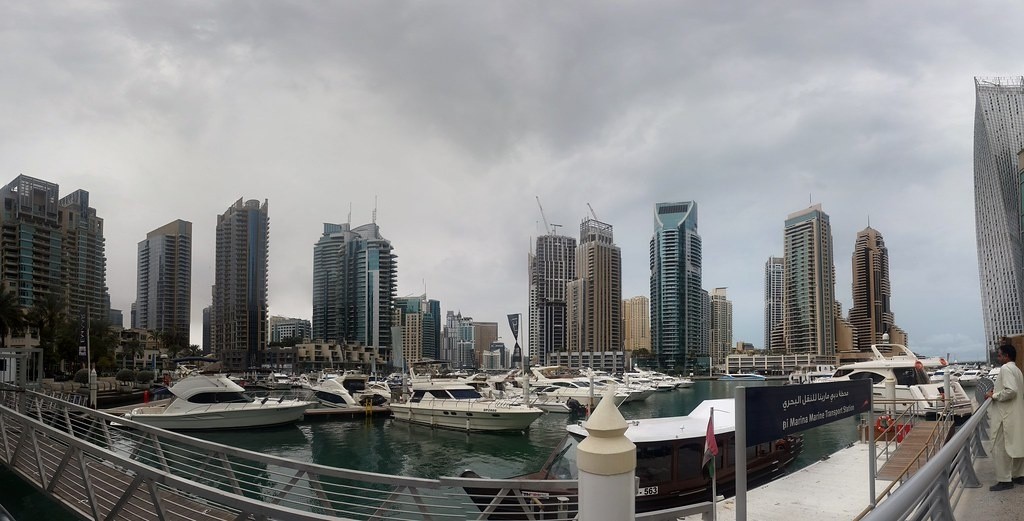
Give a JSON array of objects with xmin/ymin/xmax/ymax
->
[
  {"xmin": 717, "ymin": 372, "xmax": 769, "ymax": 382},
  {"xmin": 390, "ymin": 383, "xmax": 544, "ymax": 432},
  {"xmin": 108, "ymin": 375, "xmax": 316, "ymax": 432},
  {"xmin": 783, "ymin": 364, "xmax": 840, "ymax": 385},
  {"xmin": 809, "ymin": 343, "xmax": 974, "ymax": 423},
  {"xmin": 928, "ymin": 361, "xmax": 1001, "ymax": 388},
  {"xmin": 361, "ymin": 365, "xmax": 695, "ymax": 412},
  {"xmin": 301, "ymin": 373, "xmax": 392, "ymax": 413}
]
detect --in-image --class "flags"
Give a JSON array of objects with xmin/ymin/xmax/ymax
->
[{"xmin": 702, "ymin": 415, "xmax": 718, "ymax": 487}]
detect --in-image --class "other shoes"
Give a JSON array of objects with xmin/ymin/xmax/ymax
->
[
  {"xmin": 1011, "ymin": 477, "xmax": 1024, "ymax": 485},
  {"xmin": 989, "ymin": 482, "xmax": 1015, "ymax": 491}
]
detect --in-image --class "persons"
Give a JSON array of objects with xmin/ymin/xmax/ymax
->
[{"xmin": 985, "ymin": 344, "xmax": 1024, "ymax": 491}]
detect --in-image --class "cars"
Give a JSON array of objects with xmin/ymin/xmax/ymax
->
[{"xmin": 267, "ymin": 368, "xmax": 364, "ymax": 389}]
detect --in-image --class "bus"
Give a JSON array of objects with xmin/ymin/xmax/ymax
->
[{"xmin": 176, "ymin": 361, "xmax": 198, "ymax": 375}]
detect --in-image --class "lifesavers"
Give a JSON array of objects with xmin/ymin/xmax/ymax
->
[
  {"xmin": 877, "ymin": 415, "xmax": 893, "ymax": 432},
  {"xmin": 915, "ymin": 361, "xmax": 923, "ymax": 369},
  {"xmin": 939, "ymin": 358, "xmax": 947, "ymax": 365}
]
